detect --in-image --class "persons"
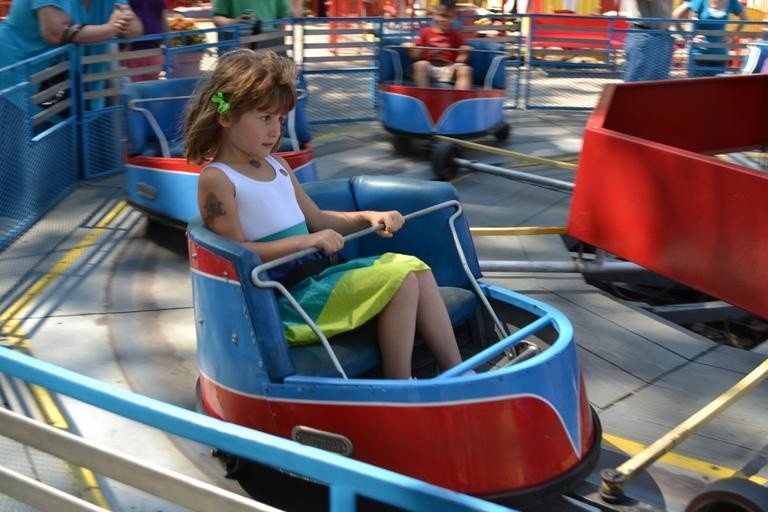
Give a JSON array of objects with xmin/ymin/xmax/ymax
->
[
  {"xmin": 184, "ymin": 50, "xmax": 465, "ymax": 379},
  {"xmin": 401, "ymin": 0, "xmax": 476, "ymax": 90},
  {"xmin": 119, "ymin": 0, "xmax": 164, "ymax": 83},
  {"xmin": 211, "ymin": 0, "xmax": 293, "ymax": 59},
  {"xmin": 671, "ymin": 0, "xmax": 747, "ymax": 78},
  {"xmin": 0, "ymin": 0, "xmax": 146, "ymax": 100},
  {"xmin": 624, "ymin": 1, "xmax": 675, "ymax": 81}
]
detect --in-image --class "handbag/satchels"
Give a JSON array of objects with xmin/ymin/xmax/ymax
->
[{"xmin": 34, "ymin": 22, "xmax": 92, "ymax": 119}]
